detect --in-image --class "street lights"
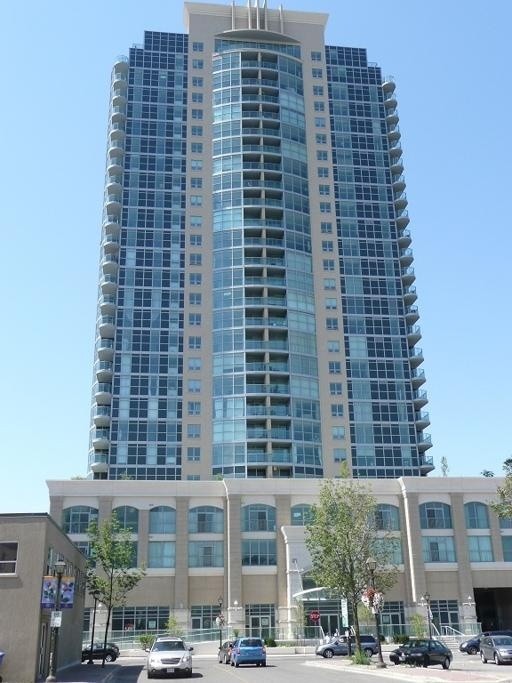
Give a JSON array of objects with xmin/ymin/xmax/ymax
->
[
  {"xmin": 46, "ymin": 557, "xmax": 66, "ymax": 681},
  {"xmin": 218, "ymin": 594, "xmax": 224, "ymax": 648},
  {"xmin": 366, "ymin": 555, "xmax": 386, "ymax": 667},
  {"xmin": 425, "ymin": 591, "xmax": 434, "ymax": 638}
]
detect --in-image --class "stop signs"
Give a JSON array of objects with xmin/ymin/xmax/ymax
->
[{"xmin": 310, "ymin": 611, "xmax": 320, "ymax": 621}]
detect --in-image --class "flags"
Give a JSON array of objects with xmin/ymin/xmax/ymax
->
[
  {"xmin": 41, "ymin": 575, "xmax": 59, "ymax": 609},
  {"xmin": 59, "ymin": 576, "xmax": 76, "ymax": 609}
]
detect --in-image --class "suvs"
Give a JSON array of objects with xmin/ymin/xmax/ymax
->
[
  {"xmin": 145, "ymin": 636, "xmax": 194, "ymax": 676},
  {"xmin": 230, "ymin": 636, "xmax": 267, "ymax": 665},
  {"xmin": 82, "ymin": 641, "xmax": 120, "ymax": 662},
  {"xmin": 316, "ymin": 633, "xmax": 378, "ymax": 656}
]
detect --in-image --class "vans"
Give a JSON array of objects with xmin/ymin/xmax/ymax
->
[{"xmin": 459, "ymin": 629, "xmax": 512, "ymax": 654}]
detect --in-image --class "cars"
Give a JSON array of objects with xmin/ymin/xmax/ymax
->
[
  {"xmin": 218, "ymin": 639, "xmax": 232, "ymax": 663},
  {"xmin": 391, "ymin": 638, "xmax": 453, "ymax": 669},
  {"xmin": 480, "ymin": 635, "xmax": 512, "ymax": 664}
]
